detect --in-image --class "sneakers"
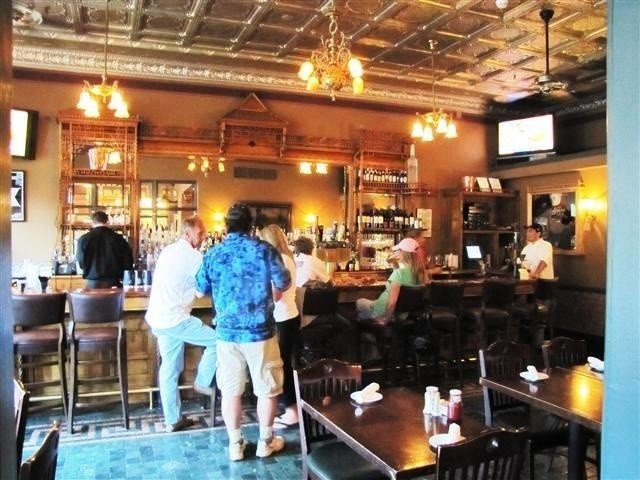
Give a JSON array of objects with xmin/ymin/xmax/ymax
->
[
  {"xmin": 256, "ymin": 432, "xmax": 284, "ymax": 457},
  {"xmin": 228, "ymin": 434, "xmax": 244, "ymax": 461},
  {"xmin": 166, "ymin": 416, "xmax": 193, "ymax": 431},
  {"xmin": 193, "ymin": 381, "xmax": 221, "ymax": 397}
]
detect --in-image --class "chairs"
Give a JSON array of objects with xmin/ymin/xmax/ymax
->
[
  {"xmin": 296, "ymin": 280, "xmax": 558, "ymax": 393},
  {"xmin": 11, "ymin": 293, "xmax": 69, "ymax": 433},
  {"xmin": 67, "ymin": 287, "xmax": 130, "ymax": 435},
  {"xmin": 11, "ymin": 378, "xmax": 31, "ymax": 480},
  {"xmin": 292, "ymin": 358, "xmax": 411, "ymax": 480},
  {"xmin": 541, "ymin": 336, "xmax": 599, "ymax": 479},
  {"xmin": 20, "ymin": 419, "xmax": 63, "ymax": 480},
  {"xmin": 478, "ymin": 339, "xmax": 591, "ymax": 480},
  {"xmin": 412, "ymin": 429, "xmax": 529, "ymax": 480}
]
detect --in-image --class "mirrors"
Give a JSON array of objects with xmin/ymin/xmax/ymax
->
[{"xmin": 57, "ymin": 142, "xmax": 358, "ymax": 259}]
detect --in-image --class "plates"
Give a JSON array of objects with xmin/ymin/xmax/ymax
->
[
  {"xmin": 350, "ymin": 391, "xmax": 383, "ymax": 406},
  {"xmin": 429, "ymin": 432, "xmax": 465, "ymax": 451},
  {"xmin": 585, "ymin": 361, "xmax": 604, "ymax": 373},
  {"xmin": 520, "ymin": 370, "xmax": 552, "ymax": 382}
]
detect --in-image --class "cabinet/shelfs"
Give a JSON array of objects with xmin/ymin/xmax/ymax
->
[
  {"xmin": 63, "ymin": 177, "xmax": 198, "ymax": 262},
  {"xmin": 440, "ymin": 187, "xmax": 522, "ymax": 270},
  {"xmin": 349, "ymin": 149, "xmax": 427, "ymax": 269},
  {"xmin": 56, "ymin": 127, "xmax": 140, "ymax": 275}
]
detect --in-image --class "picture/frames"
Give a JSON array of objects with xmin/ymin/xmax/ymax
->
[
  {"xmin": 237, "ymin": 200, "xmax": 292, "ymax": 236},
  {"xmin": 10, "ymin": 170, "xmax": 25, "ymax": 222},
  {"xmin": 526, "ymin": 189, "xmax": 583, "ymax": 256}
]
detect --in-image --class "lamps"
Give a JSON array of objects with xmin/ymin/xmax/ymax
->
[
  {"xmin": 186, "ymin": 155, "xmax": 226, "ymax": 178},
  {"xmin": 76, "ymin": 0, "xmax": 131, "ymax": 119},
  {"xmin": 298, "ymin": 0, "xmax": 365, "ymax": 102},
  {"xmin": 411, "ymin": 39, "xmax": 459, "ymax": 142}
]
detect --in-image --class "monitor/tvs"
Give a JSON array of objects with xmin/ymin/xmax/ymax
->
[
  {"xmin": 465, "ymin": 246, "xmax": 483, "ymax": 261},
  {"xmin": 496, "ymin": 112, "xmax": 558, "ymax": 159},
  {"xmin": 9, "ymin": 107, "xmax": 37, "ymax": 159}
]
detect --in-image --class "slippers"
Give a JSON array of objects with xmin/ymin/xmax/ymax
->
[{"xmin": 272, "ymin": 416, "xmax": 299, "ymax": 430}]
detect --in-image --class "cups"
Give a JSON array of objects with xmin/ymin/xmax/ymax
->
[
  {"xmin": 426, "ymin": 255, "xmax": 433, "ymax": 263},
  {"xmin": 123, "ymin": 269, "xmax": 133, "ymax": 286},
  {"xmin": 142, "ymin": 270, "xmax": 151, "ymax": 286},
  {"xmin": 434, "ymin": 254, "xmax": 443, "ymax": 266},
  {"xmin": 20, "ymin": 283, "xmax": 26, "ymax": 292},
  {"xmin": 41, "ymin": 280, "xmax": 47, "ymax": 290},
  {"xmin": 132, "ymin": 270, "xmax": 142, "ymax": 287}
]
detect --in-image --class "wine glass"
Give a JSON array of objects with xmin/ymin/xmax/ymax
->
[
  {"xmin": 361, "ymin": 233, "xmax": 395, "ymax": 248},
  {"xmin": 105, "ymin": 207, "xmax": 129, "ymax": 226},
  {"xmin": 358, "ymin": 257, "xmax": 374, "ymax": 269}
]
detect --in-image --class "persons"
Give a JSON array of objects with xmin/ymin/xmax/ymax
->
[
  {"xmin": 353, "ymin": 236, "xmax": 418, "ymax": 375},
  {"xmin": 75, "ymin": 210, "xmax": 137, "ymax": 293},
  {"xmin": 405, "ymin": 227, "xmax": 445, "ymax": 284},
  {"xmin": 256, "ymin": 217, "xmax": 304, "ymax": 435},
  {"xmin": 516, "ymin": 222, "xmax": 557, "ymax": 314},
  {"xmin": 192, "ymin": 200, "xmax": 290, "ymax": 465},
  {"xmin": 142, "ymin": 214, "xmax": 218, "ymax": 433},
  {"xmin": 290, "ymin": 233, "xmax": 341, "ymax": 331}
]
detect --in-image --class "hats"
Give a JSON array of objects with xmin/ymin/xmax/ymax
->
[
  {"xmin": 392, "ymin": 238, "xmax": 420, "ymax": 253},
  {"xmin": 227, "ymin": 204, "xmax": 251, "ymax": 220},
  {"xmin": 524, "ymin": 224, "xmax": 542, "ymax": 232}
]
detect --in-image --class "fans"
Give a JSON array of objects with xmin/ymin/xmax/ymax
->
[{"xmin": 493, "ymin": 9, "xmax": 603, "ymax": 104}]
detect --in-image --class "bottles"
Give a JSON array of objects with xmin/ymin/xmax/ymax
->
[
  {"xmin": 250, "ymin": 225, "xmax": 259, "ymax": 237},
  {"xmin": 449, "ymin": 389, "xmax": 463, "ymax": 422},
  {"xmin": 347, "ymin": 255, "xmax": 360, "ymax": 271},
  {"xmin": 203, "ymin": 230, "xmax": 225, "ymax": 246},
  {"xmin": 423, "ymin": 385, "xmax": 440, "ymax": 414},
  {"xmin": 432, "ymin": 390, "xmax": 449, "ymax": 417},
  {"xmin": 358, "ymin": 167, "xmax": 407, "ymax": 184},
  {"xmin": 286, "ymin": 214, "xmax": 345, "ymax": 243},
  {"xmin": 58, "ymin": 262, "xmax": 73, "ymax": 274},
  {"xmin": 405, "ymin": 143, "xmax": 419, "ymax": 189},
  {"xmin": 485, "ymin": 260, "xmax": 489, "ymax": 268},
  {"xmin": 355, "ymin": 206, "xmax": 422, "ymax": 230}
]
